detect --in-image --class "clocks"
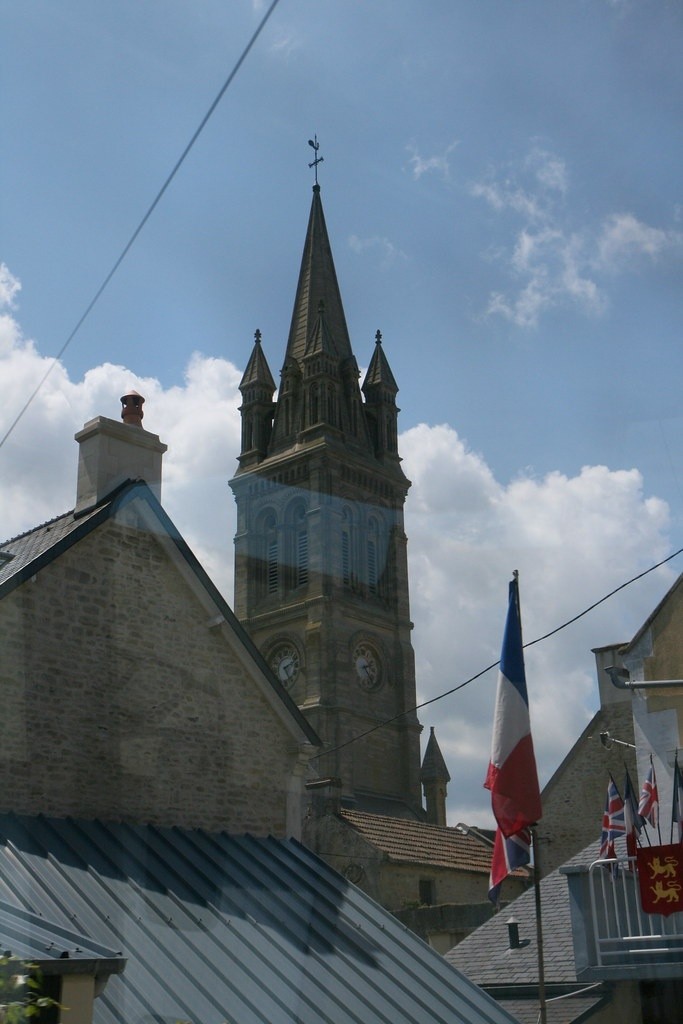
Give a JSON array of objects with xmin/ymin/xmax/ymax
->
[
  {"xmin": 352, "ymin": 641, "xmax": 384, "ymax": 691},
  {"xmin": 264, "ymin": 640, "xmax": 301, "ymax": 690}
]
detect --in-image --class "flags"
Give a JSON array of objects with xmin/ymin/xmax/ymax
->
[
  {"xmin": 482, "ymin": 577, "xmax": 542, "ymax": 908},
  {"xmin": 599, "ymin": 758, "xmax": 683, "ymax": 884}
]
